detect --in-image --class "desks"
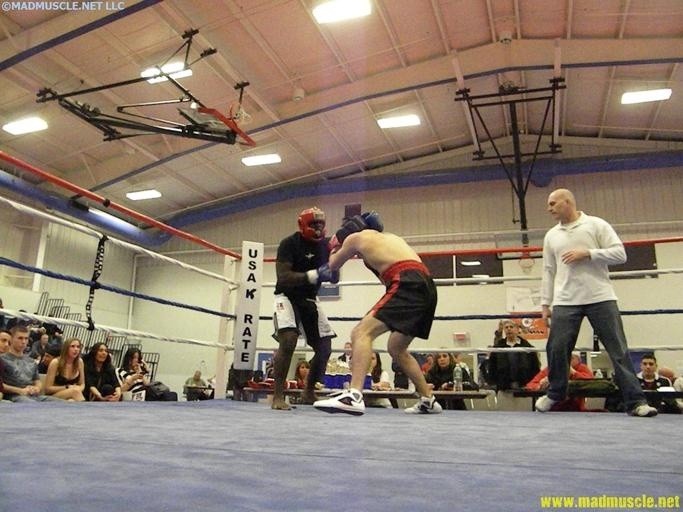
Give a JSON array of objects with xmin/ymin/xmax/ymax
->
[
  {"xmin": 513, "ymin": 387, "xmax": 683, "ymax": 413},
  {"xmin": 242, "ymin": 386, "xmax": 489, "ymax": 409},
  {"xmin": 188, "ymin": 385, "xmax": 214, "ymax": 400}
]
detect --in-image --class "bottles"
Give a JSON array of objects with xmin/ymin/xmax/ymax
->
[{"xmin": 452, "ymin": 363, "xmax": 462, "ymax": 392}]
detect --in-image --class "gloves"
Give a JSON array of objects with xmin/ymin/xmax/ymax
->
[
  {"xmin": 317, "ymin": 263, "xmax": 339, "ymax": 284},
  {"xmin": 336, "ymin": 216, "xmax": 365, "ymax": 243}
]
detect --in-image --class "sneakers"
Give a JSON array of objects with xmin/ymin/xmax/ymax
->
[
  {"xmin": 629, "ymin": 402, "xmax": 656, "ymax": 417},
  {"xmin": 536, "ymin": 393, "xmax": 558, "ymax": 414},
  {"xmin": 312, "ymin": 387, "xmax": 365, "ymax": 417},
  {"xmin": 403, "ymin": 396, "xmax": 443, "ymax": 415}
]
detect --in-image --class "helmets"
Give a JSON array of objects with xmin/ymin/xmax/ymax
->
[
  {"xmin": 361, "ymin": 210, "xmax": 384, "ymax": 233},
  {"xmin": 298, "ymin": 205, "xmax": 326, "ymax": 241}
]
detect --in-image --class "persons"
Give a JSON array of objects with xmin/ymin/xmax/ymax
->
[
  {"xmin": 330, "ymin": 210, "xmax": 442, "ymax": 416},
  {"xmin": 271, "ymin": 207, "xmax": 340, "ymax": 410},
  {"xmin": 0, "ymin": 310, "xmax": 683, "ymax": 414},
  {"xmin": 535, "ymin": 189, "xmax": 657, "ymax": 416}
]
